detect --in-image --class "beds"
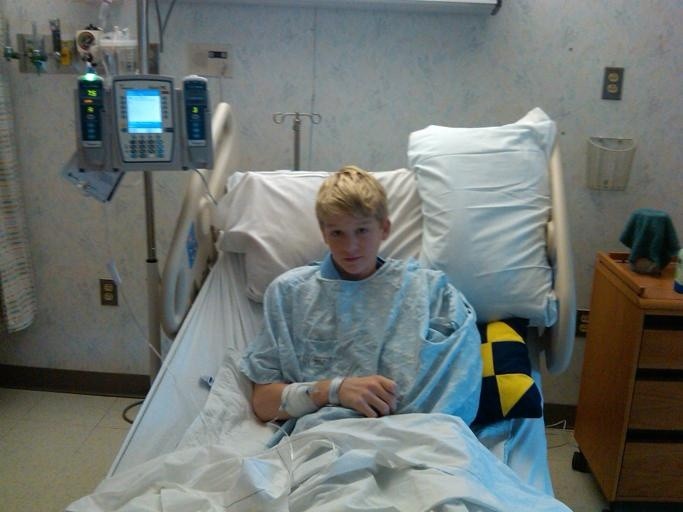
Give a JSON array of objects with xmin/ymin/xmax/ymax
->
[{"xmin": 97, "ymin": 101, "xmax": 575, "ymax": 512}]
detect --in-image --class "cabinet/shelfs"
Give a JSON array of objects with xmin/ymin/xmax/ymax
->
[{"xmin": 572, "ymin": 251, "xmax": 683, "ymax": 507}]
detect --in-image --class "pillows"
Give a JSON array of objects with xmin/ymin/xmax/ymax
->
[{"xmin": 464, "ymin": 318, "xmax": 544, "ymax": 423}]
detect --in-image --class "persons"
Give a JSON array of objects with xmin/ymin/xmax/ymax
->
[{"xmin": 234, "ymin": 164, "xmax": 482, "ymax": 426}]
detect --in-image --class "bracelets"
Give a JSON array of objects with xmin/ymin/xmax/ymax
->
[{"xmin": 327, "ymin": 376, "xmax": 346, "ymax": 407}]
